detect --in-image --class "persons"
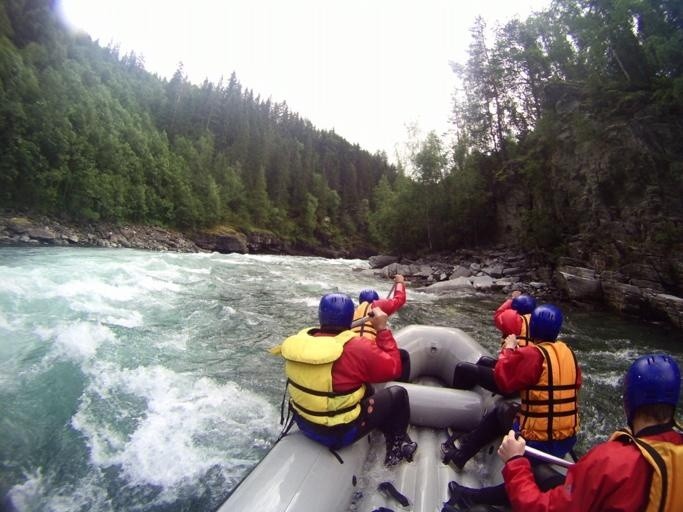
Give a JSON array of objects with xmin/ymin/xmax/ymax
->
[
  {"xmin": 277, "ymin": 291, "xmax": 418, "ymax": 467},
  {"xmin": 440, "ymin": 302, "xmax": 580, "ymax": 471},
  {"xmin": 441, "ymin": 347, "xmax": 683, "ymax": 512},
  {"xmin": 349, "ymin": 272, "xmax": 412, "ymax": 383},
  {"xmin": 445, "ymin": 290, "xmax": 535, "ymax": 400}
]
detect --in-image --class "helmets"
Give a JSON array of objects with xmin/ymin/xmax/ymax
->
[
  {"xmin": 512, "ymin": 295, "xmax": 562, "ymax": 339},
  {"xmin": 623, "ymin": 355, "xmax": 680, "ymax": 426},
  {"xmin": 319, "ymin": 294, "xmax": 353, "ymax": 326},
  {"xmin": 359, "ymin": 290, "xmax": 378, "ymax": 304}
]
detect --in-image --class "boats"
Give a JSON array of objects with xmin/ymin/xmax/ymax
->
[{"xmin": 212, "ymin": 323, "xmax": 580, "ymax": 511}]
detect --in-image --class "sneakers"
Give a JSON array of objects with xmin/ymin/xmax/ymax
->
[
  {"xmin": 440, "ymin": 443, "xmax": 466, "ymax": 473},
  {"xmin": 449, "ymin": 481, "xmax": 473, "ymax": 510}
]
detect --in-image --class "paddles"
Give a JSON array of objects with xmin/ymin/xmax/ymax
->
[{"xmin": 269, "ymin": 312, "xmax": 374, "ymax": 355}]
